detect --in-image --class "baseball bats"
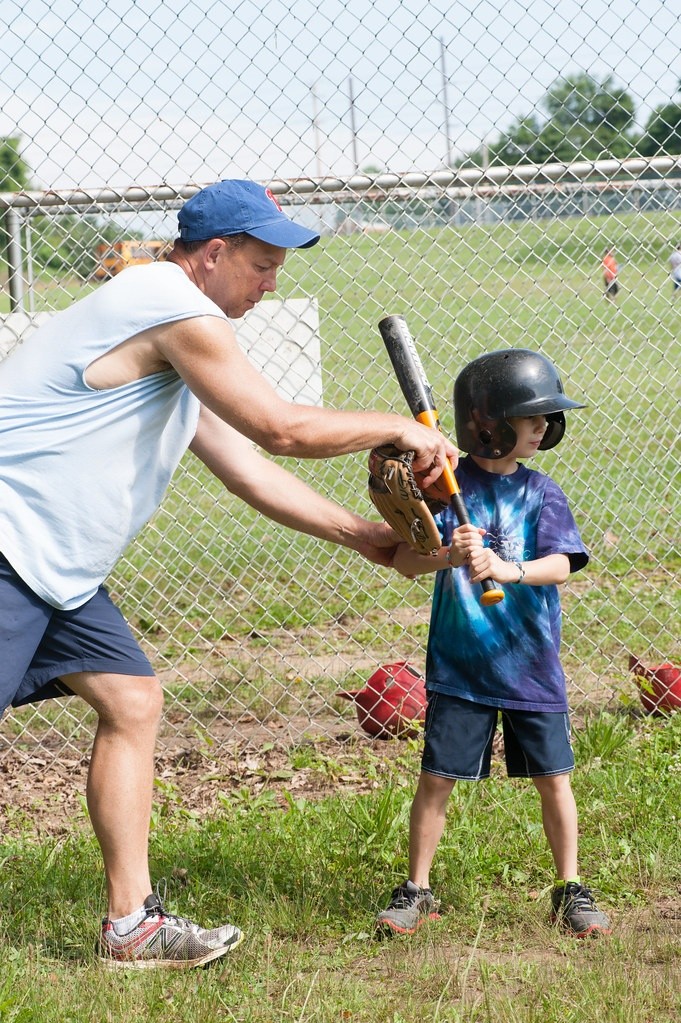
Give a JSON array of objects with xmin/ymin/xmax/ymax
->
[{"xmin": 377, "ymin": 318, "xmax": 505, "ymax": 607}]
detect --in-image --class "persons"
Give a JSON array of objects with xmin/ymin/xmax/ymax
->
[
  {"xmin": 669, "ymin": 243, "xmax": 681, "ymax": 299},
  {"xmin": 0, "ymin": 179, "xmax": 459, "ymax": 973},
  {"xmin": 601, "ymin": 247, "xmax": 619, "ymax": 305},
  {"xmin": 375, "ymin": 348, "xmax": 612, "ymax": 942}
]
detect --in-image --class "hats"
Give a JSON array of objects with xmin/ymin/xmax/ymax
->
[{"xmin": 177, "ymin": 179, "xmax": 319, "ymax": 249}]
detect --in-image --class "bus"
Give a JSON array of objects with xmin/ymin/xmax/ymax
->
[{"xmin": 96, "ymin": 240, "xmax": 175, "ymax": 278}]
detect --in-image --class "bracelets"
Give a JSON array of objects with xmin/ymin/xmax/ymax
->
[
  {"xmin": 445, "ymin": 548, "xmax": 458, "ymax": 568},
  {"xmin": 512, "ymin": 562, "xmax": 525, "ymax": 585}
]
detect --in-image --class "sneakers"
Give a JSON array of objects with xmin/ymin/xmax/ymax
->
[
  {"xmin": 93, "ymin": 894, "xmax": 243, "ymax": 970},
  {"xmin": 376, "ymin": 880, "xmax": 441, "ymax": 933},
  {"xmin": 549, "ymin": 882, "xmax": 611, "ymax": 938}
]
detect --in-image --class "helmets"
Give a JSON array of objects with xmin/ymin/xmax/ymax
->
[{"xmin": 454, "ymin": 349, "xmax": 585, "ymax": 459}]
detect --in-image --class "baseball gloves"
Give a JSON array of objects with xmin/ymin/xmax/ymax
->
[{"xmin": 365, "ymin": 445, "xmax": 441, "ymax": 559}]
